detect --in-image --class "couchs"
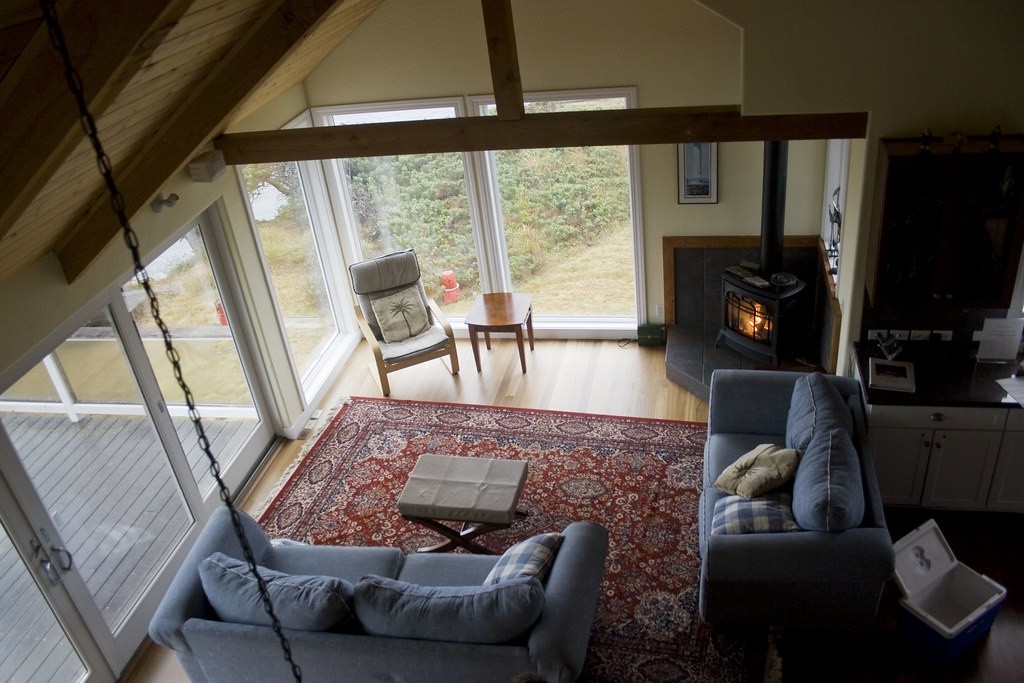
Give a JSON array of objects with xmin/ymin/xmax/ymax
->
[
  {"xmin": 148, "ymin": 505, "xmax": 610, "ymax": 683},
  {"xmin": 698, "ymin": 370, "xmax": 890, "ymax": 635}
]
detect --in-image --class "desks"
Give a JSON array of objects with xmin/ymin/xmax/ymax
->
[{"xmin": 465, "ymin": 291, "xmax": 534, "ymax": 374}]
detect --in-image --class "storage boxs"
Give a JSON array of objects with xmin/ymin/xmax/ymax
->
[{"xmin": 890, "ymin": 517, "xmax": 1007, "ymax": 668}]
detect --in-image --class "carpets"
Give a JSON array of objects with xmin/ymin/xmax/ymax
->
[{"xmin": 256, "ymin": 397, "xmax": 779, "ymax": 683}]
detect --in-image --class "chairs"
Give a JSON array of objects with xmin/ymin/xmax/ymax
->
[{"xmin": 349, "ymin": 247, "xmax": 459, "ymax": 396}]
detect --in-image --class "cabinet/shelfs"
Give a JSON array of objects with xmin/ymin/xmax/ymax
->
[{"xmin": 862, "ymin": 405, "xmax": 1024, "ymax": 513}]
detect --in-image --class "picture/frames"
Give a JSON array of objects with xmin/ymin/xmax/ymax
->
[
  {"xmin": 865, "ymin": 137, "xmax": 1024, "ymax": 309},
  {"xmin": 676, "ymin": 142, "xmax": 719, "ymax": 206}
]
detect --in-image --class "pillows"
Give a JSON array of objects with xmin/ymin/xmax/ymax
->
[
  {"xmin": 715, "ymin": 443, "xmax": 802, "ymax": 503},
  {"xmin": 710, "ymin": 494, "xmax": 798, "ymax": 536},
  {"xmin": 483, "ymin": 530, "xmax": 562, "ymax": 584},
  {"xmin": 198, "ymin": 550, "xmax": 351, "ymax": 634},
  {"xmin": 785, "ymin": 373, "xmax": 852, "ymax": 444},
  {"xmin": 353, "ymin": 574, "xmax": 542, "ymax": 646},
  {"xmin": 793, "ymin": 430, "xmax": 866, "ymax": 530},
  {"xmin": 370, "ymin": 285, "xmax": 433, "ymax": 342}
]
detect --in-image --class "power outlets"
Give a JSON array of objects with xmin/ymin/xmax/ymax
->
[
  {"xmin": 868, "ymin": 330, "xmax": 887, "ymax": 340},
  {"xmin": 890, "ymin": 331, "xmax": 909, "ymax": 340},
  {"xmin": 911, "ymin": 331, "xmax": 930, "ymax": 341},
  {"xmin": 933, "ymin": 331, "xmax": 952, "ymax": 341}
]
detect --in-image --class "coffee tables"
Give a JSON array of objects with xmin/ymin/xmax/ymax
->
[{"xmin": 399, "ymin": 453, "xmax": 529, "ymax": 556}]
